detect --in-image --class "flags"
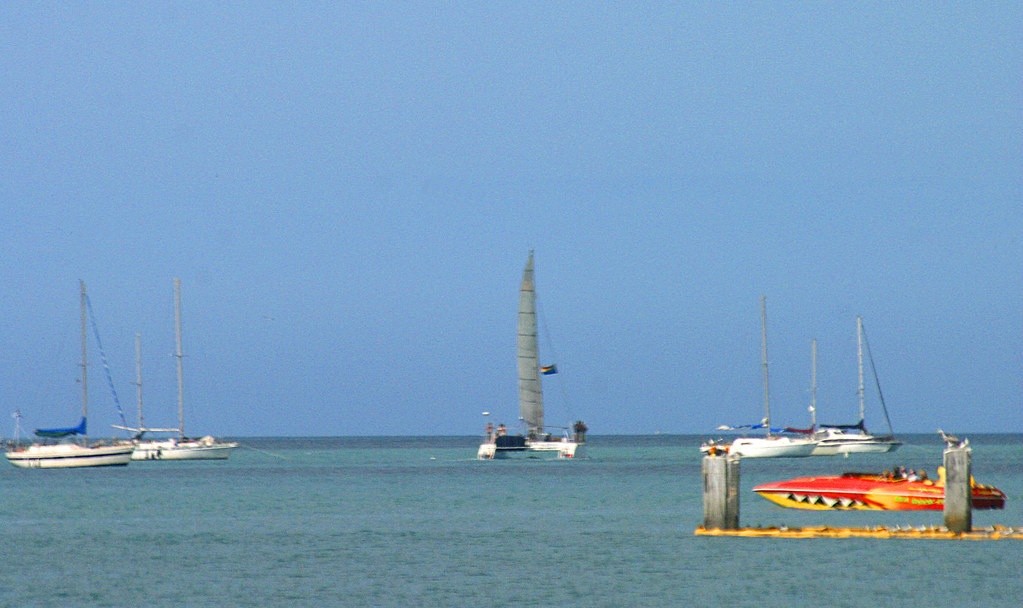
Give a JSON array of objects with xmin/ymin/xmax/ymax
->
[{"xmin": 539, "ymin": 364, "xmax": 559, "ymax": 375}]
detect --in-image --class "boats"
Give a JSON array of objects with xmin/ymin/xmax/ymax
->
[
  {"xmin": 752, "ymin": 462, "xmax": 1006, "ymax": 512},
  {"xmin": 476, "ymin": 409, "xmax": 579, "ymax": 463}
]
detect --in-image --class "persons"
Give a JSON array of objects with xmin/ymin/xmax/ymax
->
[
  {"xmin": 488, "ymin": 423, "xmax": 506, "ymax": 442},
  {"xmin": 574, "ymin": 421, "xmax": 586, "ymax": 442},
  {"xmin": 879, "ymin": 465, "xmax": 928, "ymax": 483},
  {"xmin": 136, "ymin": 431, "xmax": 146, "ymax": 440},
  {"xmin": 529, "ymin": 430, "xmax": 553, "ymax": 441}
]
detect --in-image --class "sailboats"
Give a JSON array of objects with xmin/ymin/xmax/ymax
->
[
  {"xmin": 3, "ymin": 279, "xmax": 139, "ymax": 469},
  {"xmin": 700, "ymin": 292, "xmax": 904, "ymax": 464},
  {"xmin": 116, "ymin": 276, "xmax": 240, "ymax": 461}
]
